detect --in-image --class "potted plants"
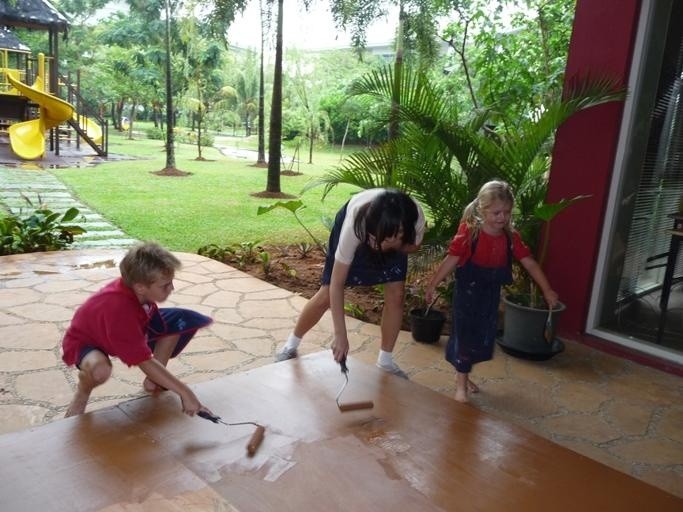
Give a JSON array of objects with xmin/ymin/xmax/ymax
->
[
  {"xmin": 408, "ymin": 280, "xmax": 455, "ymax": 344},
  {"xmin": 298, "ymin": 56, "xmax": 633, "ymax": 360}
]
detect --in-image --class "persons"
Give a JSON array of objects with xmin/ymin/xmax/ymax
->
[
  {"xmin": 60, "ymin": 239, "xmax": 222, "ymax": 424},
  {"xmin": 423, "ymin": 179, "xmax": 560, "ymax": 405},
  {"xmin": 273, "ymin": 186, "xmax": 427, "ymax": 380}
]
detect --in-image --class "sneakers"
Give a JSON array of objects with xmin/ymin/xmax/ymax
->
[
  {"xmin": 274, "ymin": 348, "xmax": 297, "ymax": 363},
  {"xmin": 376, "ymin": 361, "xmax": 412, "ymax": 385}
]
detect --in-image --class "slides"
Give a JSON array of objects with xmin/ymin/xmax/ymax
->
[
  {"xmin": 73, "ymin": 112, "xmax": 102, "ymax": 144},
  {"xmin": 7, "ymin": 71, "xmax": 75, "ymax": 160}
]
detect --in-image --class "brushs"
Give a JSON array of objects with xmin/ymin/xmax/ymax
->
[
  {"xmin": 543, "ymin": 306, "xmax": 555, "ymax": 342},
  {"xmin": 334, "ymin": 356, "xmax": 375, "ymax": 411},
  {"xmin": 197, "ymin": 411, "xmax": 265, "ymax": 454}
]
